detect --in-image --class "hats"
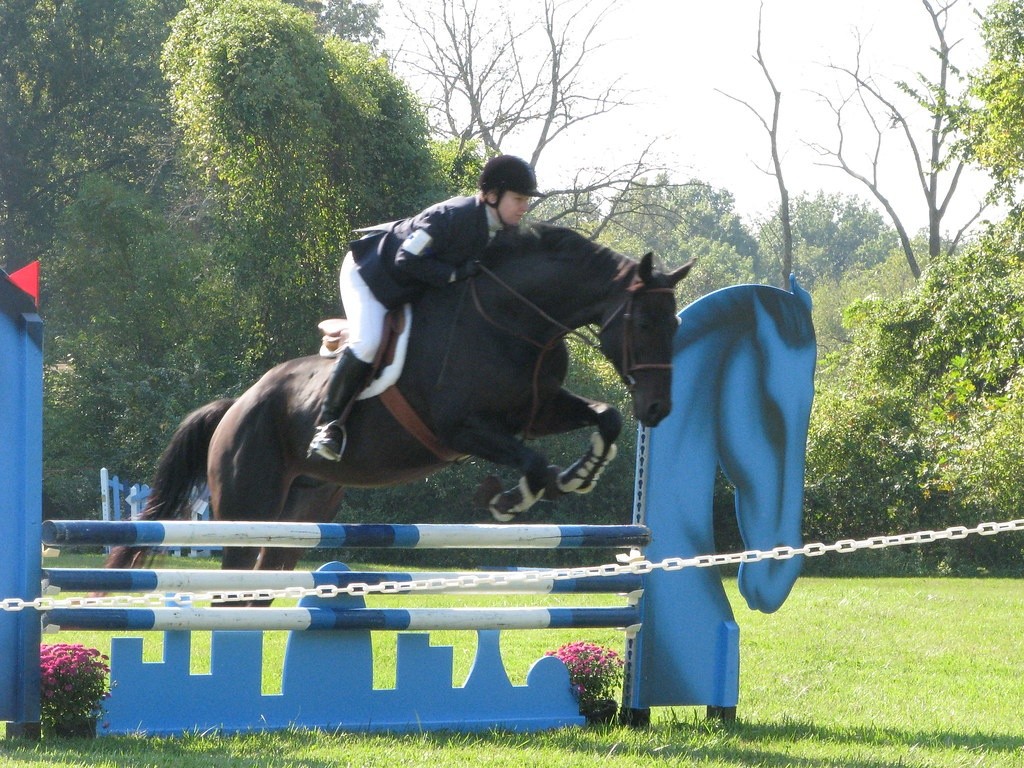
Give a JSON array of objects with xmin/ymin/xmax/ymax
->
[{"xmin": 478, "ymin": 155, "xmax": 547, "ymax": 198}]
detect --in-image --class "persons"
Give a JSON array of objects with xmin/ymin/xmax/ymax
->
[{"xmin": 308, "ymin": 155, "xmax": 546, "ymax": 463}]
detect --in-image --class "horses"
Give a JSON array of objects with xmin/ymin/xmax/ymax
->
[
  {"xmin": 84, "ymin": 219, "xmax": 699, "ymax": 608},
  {"xmin": 617, "ymin": 272, "xmax": 817, "ymax": 706}
]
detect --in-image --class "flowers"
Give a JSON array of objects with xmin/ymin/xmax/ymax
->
[
  {"xmin": 543, "ymin": 641, "xmax": 629, "ymax": 713},
  {"xmin": 40, "ymin": 642, "xmax": 112, "ymax": 735}
]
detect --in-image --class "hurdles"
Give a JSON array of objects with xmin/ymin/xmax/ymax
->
[{"xmin": 0, "ymin": 270, "xmax": 817, "ymax": 747}]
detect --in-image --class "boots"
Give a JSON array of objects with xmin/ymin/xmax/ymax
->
[{"xmin": 307, "ymin": 347, "xmax": 384, "ymax": 463}]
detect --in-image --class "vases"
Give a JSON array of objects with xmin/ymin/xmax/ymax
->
[
  {"xmin": 581, "ymin": 700, "xmax": 619, "ymax": 724},
  {"xmin": 53, "ymin": 719, "xmax": 96, "ymax": 738}
]
